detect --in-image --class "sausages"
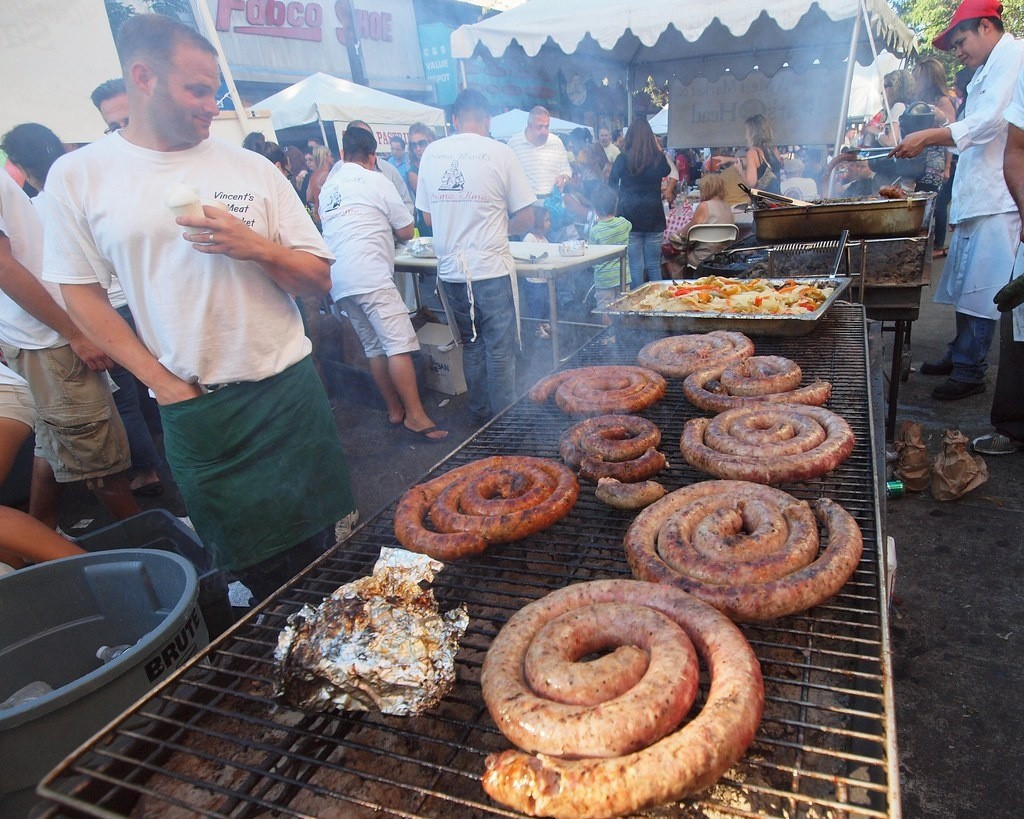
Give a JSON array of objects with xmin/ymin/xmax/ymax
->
[
  {"xmin": 623, "ymin": 479, "xmax": 863, "ymax": 622},
  {"xmin": 528, "ymin": 365, "xmax": 666, "ymax": 417},
  {"xmin": 394, "ymin": 455, "xmax": 578, "ymax": 558},
  {"xmin": 480, "ymin": 577, "xmax": 764, "ymax": 819},
  {"xmin": 558, "ymin": 414, "xmax": 670, "ymax": 482},
  {"xmin": 680, "ymin": 403, "xmax": 855, "ymax": 484},
  {"xmin": 595, "ymin": 477, "xmax": 668, "ymax": 509},
  {"xmin": 637, "ymin": 330, "xmax": 754, "ymax": 379},
  {"xmin": 879, "ymin": 185, "xmax": 910, "ymax": 198},
  {"xmin": 682, "ymin": 355, "xmax": 832, "ymax": 412},
  {"xmin": 821, "ymin": 152, "xmax": 857, "ymax": 182}
]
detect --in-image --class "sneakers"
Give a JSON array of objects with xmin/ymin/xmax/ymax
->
[{"xmin": 971, "ymin": 432, "xmax": 1024, "ymax": 453}]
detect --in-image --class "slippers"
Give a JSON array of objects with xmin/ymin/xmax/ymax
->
[
  {"xmin": 401, "ymin": 424, "xmax": 450, "ymax": 442},
  {"xmin": 387, "ymin": 410, "xmax": 405, "ymax": 428}
]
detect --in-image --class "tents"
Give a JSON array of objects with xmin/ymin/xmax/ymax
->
[
  {"xmin": 245, "ymin": 72, "xmax": 447, "ymax": 167},
  {"xmin": 489, "ymin": 108, "xmax": 596, "ymax": 143},
  {"xmin": 450, "ymin": 0, "xmax": 921, "ymax": 204},
  {"xmin": 623, "ymin": 102, "xmax": 669, "ymax": 141}
]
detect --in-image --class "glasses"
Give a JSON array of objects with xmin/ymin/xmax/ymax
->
[
  {"xmin": 281, "ymin": 165, "xmax": 293, "ymax": 180},
  {"xmin": 104, "ymin": 120, "xmax": 130, "ymax": 135},
  {"xmin": 411, "ymin": 139, "xmax": 430, "ymax": 149},
  {"xmin": 884, "ymin": 85, "xmax": 889, "ymax": 89}
]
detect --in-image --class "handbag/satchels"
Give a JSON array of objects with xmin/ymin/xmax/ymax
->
[{"xmin": 754, "ymin": 148, "xmax": 776, "ymax": 190}]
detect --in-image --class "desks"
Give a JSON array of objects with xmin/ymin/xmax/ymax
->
[{"xmin": 391, "ymin": 238, "xmax": 627, "ymax": 368}]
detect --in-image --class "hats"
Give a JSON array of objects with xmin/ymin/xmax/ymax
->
[
  {"xmin": 783, "ymin": 159, "xmax": 802, "ymax": 172},
  {"xmin": 932, "ymin": 0, "xmax": 1003, "ymax": 51}
]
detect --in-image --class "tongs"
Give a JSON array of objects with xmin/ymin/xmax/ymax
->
[
  {"xmin": 747, "ymin": 189, "xmax": 813, "ymax": 207},
  {"xmin": 847, "ymin": 147, "xmax": 894, "ymax": 162},
  {"xmin": 831, "ymin": 229, "xmax": 853, "ymax": 304}
]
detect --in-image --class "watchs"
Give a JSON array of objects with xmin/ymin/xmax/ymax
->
[
  {"xmin": 874, "ymin": 132, "xmax": 885, "ymax": 140},
  {"xmin": 559, "ymin": 175, "xmax": 566, "ymax": 184}
]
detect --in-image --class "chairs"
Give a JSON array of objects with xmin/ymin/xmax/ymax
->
[{"xmin": 683, "ymin": 223, "xmax": 741, "ymax": 277}]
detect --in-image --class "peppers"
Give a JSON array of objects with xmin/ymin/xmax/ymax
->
[{"xmin": 660, "ymin": 275, "xmax": 826, "ymax": 314}]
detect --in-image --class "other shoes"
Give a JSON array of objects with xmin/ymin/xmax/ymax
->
[
  {"xmin": 932, "ymin": 379, "xmax": 986, "ymax": 401},
  {"xmin": 921, "ymin": 360, "xmax": 951, "ymax": 373}
]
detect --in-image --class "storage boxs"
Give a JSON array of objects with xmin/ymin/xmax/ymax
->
[
  {"xmin": 316, "ymin": 337, "xmax": 425, "ymax": 416},
  {"xmin": 416, "ymin": 322, "xmax": 472, "ymax": 398},
  {"xmin": 64, "ymin": 508, "xmax": 233, "ymax": 642}
]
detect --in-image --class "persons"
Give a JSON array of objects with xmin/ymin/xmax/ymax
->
[
  {"xmin": 589, "ymin": 184, "xmax": 632, "ymax": 345},
  {"xmin": 711, "ymin": 114, "xmax": 786, "ymax": 230},
  {"xmin": 0, "ymin": 59, "xmax": 974, "ymax": 577},
  {"xmin": 415, "ymin": 89, "xmax": 537, "ymax": 449},
  {"xmin": 41, "ymin": 14, "xmax": 358, "ymax": 606},
  {"xmin": 971, "ymin": 58, "xmax": 1024, "ymax": 454},
  {"xmin": 885, "ymin": 0, "xmax": 1024, "ymax": 404},
  {"xmin": 667, "ymin": 173, "xmax": 734, "ymax": 281},
  {"xmin": 504, "ymin": 107, "xmax": 573, "ymax": 307},
  {"xmin": 318, "ymin": 125, "xmax": 450, "ymax": 442},
  {"xmin": 608, "ymin": 116, "xmax": 672, "ymax": 289}
]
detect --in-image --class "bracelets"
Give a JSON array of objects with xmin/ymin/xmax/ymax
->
[{"xmin": 739, "ymin": 170, "xmax": 742, "ymax": 176}]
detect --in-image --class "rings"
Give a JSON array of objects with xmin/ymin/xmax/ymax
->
[{"xmin": 209, "ymin": 233, "xmax": 214, "ymax": 242}]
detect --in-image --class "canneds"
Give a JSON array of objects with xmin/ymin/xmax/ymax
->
[{"xmin": 887, "ymin": 480, "xmax": 905, "ymax": 498}]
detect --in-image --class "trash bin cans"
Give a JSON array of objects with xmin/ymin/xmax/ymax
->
[{"xmin": 0, "ymin": 548, "xmax": 212, "ymax": 819}]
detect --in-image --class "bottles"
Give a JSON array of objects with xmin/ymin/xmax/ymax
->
[
  {"xmin": 95, "ymin": 645, "xmax": 131, "ymax": 663},
  {"xmin": 0, "ymin": 681, "xmax": 52, "ymax": 714}
]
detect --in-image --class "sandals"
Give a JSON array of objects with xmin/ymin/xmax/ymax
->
[
  {"xmin": 932, "ymin": 245, "xmax": 949, "ymax": 258},
  {"xmin": 130, "ymin": 477, "xmax": 162, "ymax": 495}
]
